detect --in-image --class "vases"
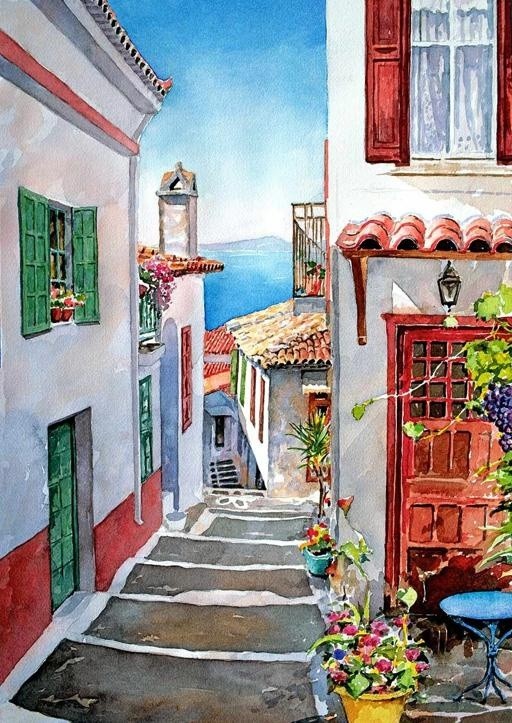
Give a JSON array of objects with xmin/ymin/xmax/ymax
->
[
  {"xmin": 304, "ymin": 548, "xmax": 332, "ymax": 577},
  {"xmin": 332, "ymin": 682, "xmax": 410, "ymax": 723},
  {"xmin": 51, "ymin": 307, "xmax": 74, "ymax": 322}
]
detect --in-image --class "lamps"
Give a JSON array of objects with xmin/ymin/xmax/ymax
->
[{"xmin": 437, "ymin": 260, "xmax": 462, "ymax": 314}]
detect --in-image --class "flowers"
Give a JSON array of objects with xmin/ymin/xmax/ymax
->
[
  {"xmin": 300, "ymin": 520, "xmax": 339, "ymax": 550},
  {"xmin": 308, "ymin": 599, "xmax": 428, "ymax": 698},
  {"xmin": 50, "ymin": 286, "xmax": 86, "ymax": 306},
  {"xmin": 136, "ymin": 253, "xmax": 178, "ymax": 312}
]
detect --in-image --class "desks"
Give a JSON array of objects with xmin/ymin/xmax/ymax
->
[{"xmin": 438, "ymin": 589, "xmax": 512, "ymax": 706}]
